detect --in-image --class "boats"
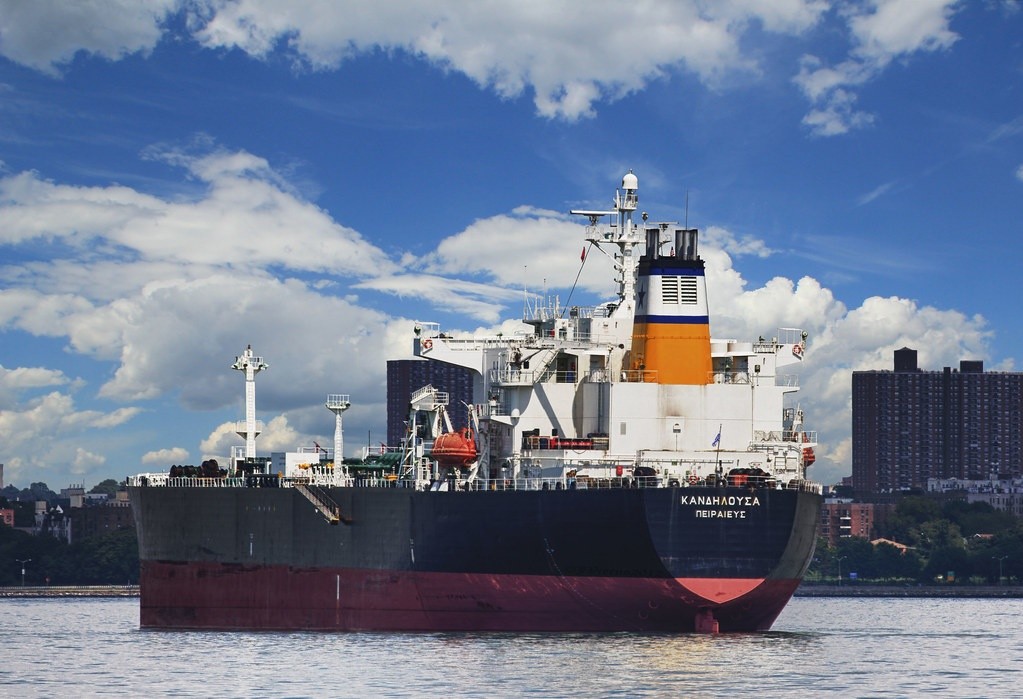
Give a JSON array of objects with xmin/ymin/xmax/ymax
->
[{"xmin": 132, "ymin": 169, "xmax": 844, "ymax": 645}]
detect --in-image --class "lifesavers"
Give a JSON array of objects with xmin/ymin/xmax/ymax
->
[
  {"xmin": 793, "ymin": 345, "xmax": 801, "ymax": 354},
  {"xmin": 424, "ymin": 340, "xmax": 433, "ymax": 348}
]
